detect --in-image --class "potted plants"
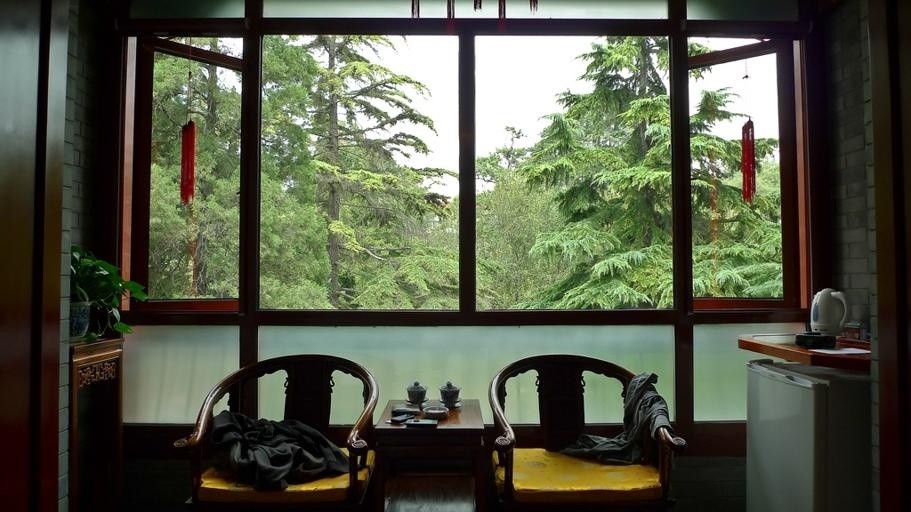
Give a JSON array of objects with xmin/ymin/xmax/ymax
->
[{"xmin": 71, "ymin": 245, "xmax": 149, "ymax": 342}]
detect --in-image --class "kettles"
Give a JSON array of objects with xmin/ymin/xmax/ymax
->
[{"xmin": 811, "ymin": 287, "xmax": 848, "ymax": 337}]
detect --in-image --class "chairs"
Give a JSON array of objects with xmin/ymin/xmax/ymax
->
[
  {"xmin": 488, "ymin": 352, "xmax": 689, "ymax": 512},
  {"xmin": 172, "ymin": 354, "xmax": 379, "ymax": 512}
]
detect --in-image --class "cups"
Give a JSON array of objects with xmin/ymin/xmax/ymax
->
[
  {"xmin": 850, "ymin": 305, "xmax": 865, "ymax": 321},
  {"xmin": 406, "ymin": 382, "xmax": 424, "ymax": 401},
  {"xmin": 439, "ymin": 382, "xmax": 461, "ymax": 403}
]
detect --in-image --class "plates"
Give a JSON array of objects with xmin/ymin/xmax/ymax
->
[
  {"xmin": 406, "ymin": 399, "xmax": 428, "ymax": 404},
  {"xmin": 439, "ymin": 400, "xmax": 461, "ymax": 406}
]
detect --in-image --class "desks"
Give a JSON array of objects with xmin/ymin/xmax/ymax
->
[
  {"xmin": 67, "ymin": 337, "xmax": 127, "ymax": 512},
  {"xmin": 375, "ymin": 399, "xmax": 486, "ymax": 512}
]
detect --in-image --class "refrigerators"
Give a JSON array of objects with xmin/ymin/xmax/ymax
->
[{"xmin": 744, "ymin": 360, "xmax": 872, "ymax": 512}]
